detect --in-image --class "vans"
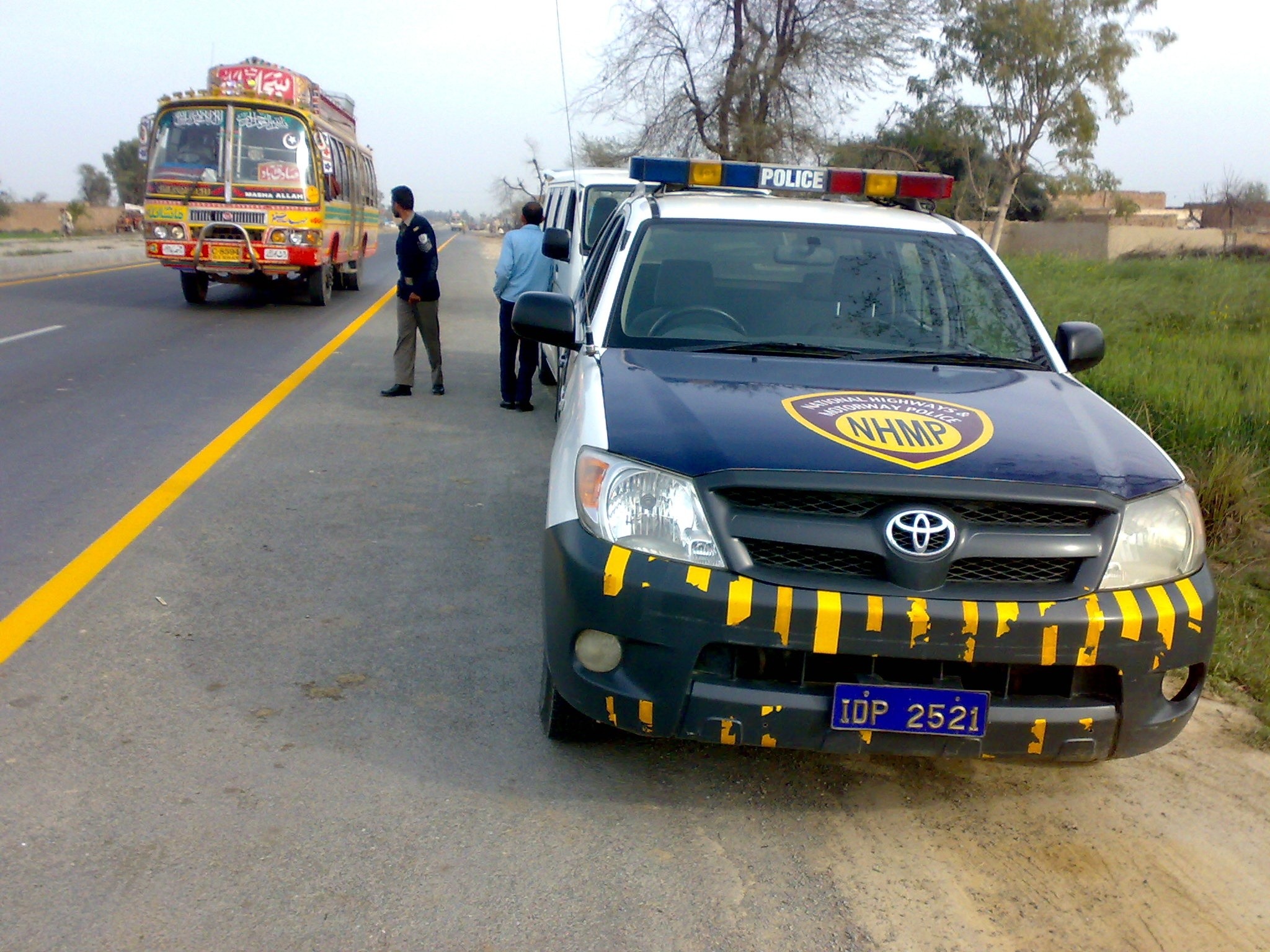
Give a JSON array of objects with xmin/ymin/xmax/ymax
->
[{"xmin": 534, "ymin": 170, "xmax": 799, "ymax": 384}]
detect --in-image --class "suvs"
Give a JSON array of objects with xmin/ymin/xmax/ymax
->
[{"xmin": 510, "ymin": 157, "xmax": 1218, "ymax": 763}]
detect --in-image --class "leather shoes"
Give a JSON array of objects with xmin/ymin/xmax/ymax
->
[
  {"xmin": 431, "ymin": 383, "xmax": 446, "ymax": 395},
  {"xmin": 380, "ymin": 383, "xmax": 412, "ymax": 396},
  {"xmin": 516, "ymin": 402, "xmax": 533, "ymax": 411},
  {"xmin": 500, "ymin": 401, "xmax": 517, "ymax": 410}
]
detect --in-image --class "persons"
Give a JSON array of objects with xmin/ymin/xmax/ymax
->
[
  {"xmin": 125, "ymin": 214, "xmax": 144, "ymax": 233},
  {"xmin": 492, "ymin": 202, "xmax": 555, "ymax": 412},
  {"xmin": 380, "ymin": 185, "xmax": 445, "ymax": 396},
  {"xmin": 57, "ymin": 207, "xmax": 72, "ymax": 237}
]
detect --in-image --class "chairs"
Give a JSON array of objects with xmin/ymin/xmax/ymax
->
[{"xmin": 636, "ymin": 254, "xmax": 920, "ymax": 346}]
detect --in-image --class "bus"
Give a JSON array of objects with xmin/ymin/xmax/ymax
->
[
  {"xmin": 450, "ymin": 213, "xmax": 463, "ymax": 231},
  {"xmin": 138, "ymin": 57, "xmax": 380, "ymax": 306}
]
